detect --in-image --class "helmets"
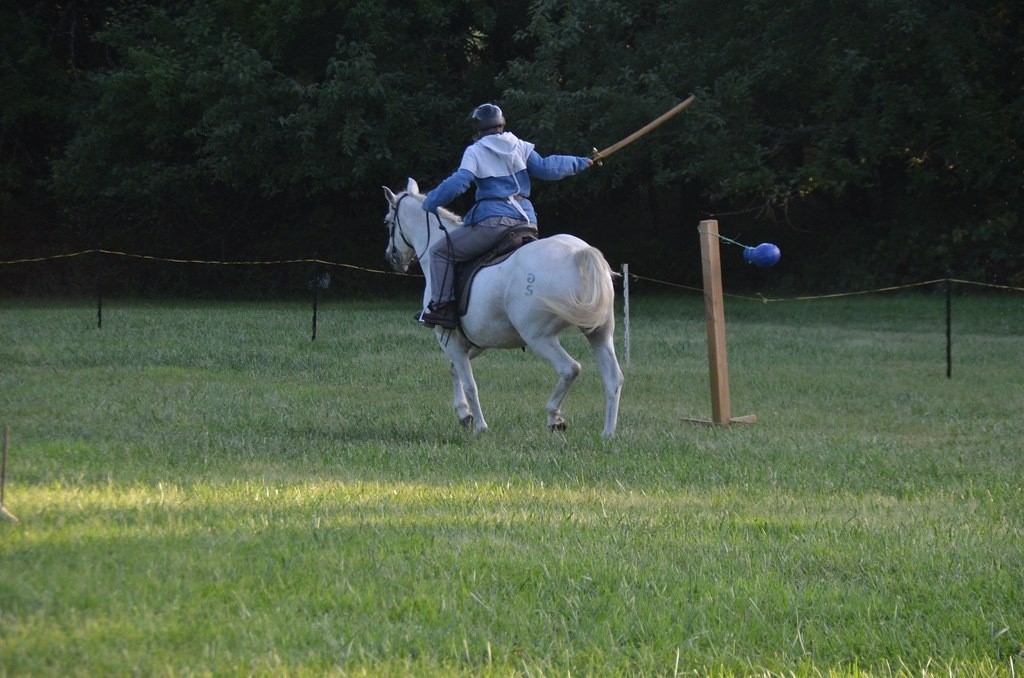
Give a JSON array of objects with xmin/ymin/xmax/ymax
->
[{"xmin": 470, "ymin": 104, "xmax": 506, "ymax": 130}]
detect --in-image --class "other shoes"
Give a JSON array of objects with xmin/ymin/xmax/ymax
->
[{"xmin": 414, "ymin": 311, "xmax": 457, "ymax": 329}]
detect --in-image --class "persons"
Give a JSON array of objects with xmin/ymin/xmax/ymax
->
[{"xmin": 413, "ymin": 104, "xmax": 593, "ymax": 330}]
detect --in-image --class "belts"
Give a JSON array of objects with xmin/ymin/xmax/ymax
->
[{"xmin": 481, "ymin": 192, "xmax": 524, "ymax": 200}]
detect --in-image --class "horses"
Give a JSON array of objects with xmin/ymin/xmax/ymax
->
[{"xmin": 381, "ymin": 176, "xmax": 626, "ymax": 438}]
